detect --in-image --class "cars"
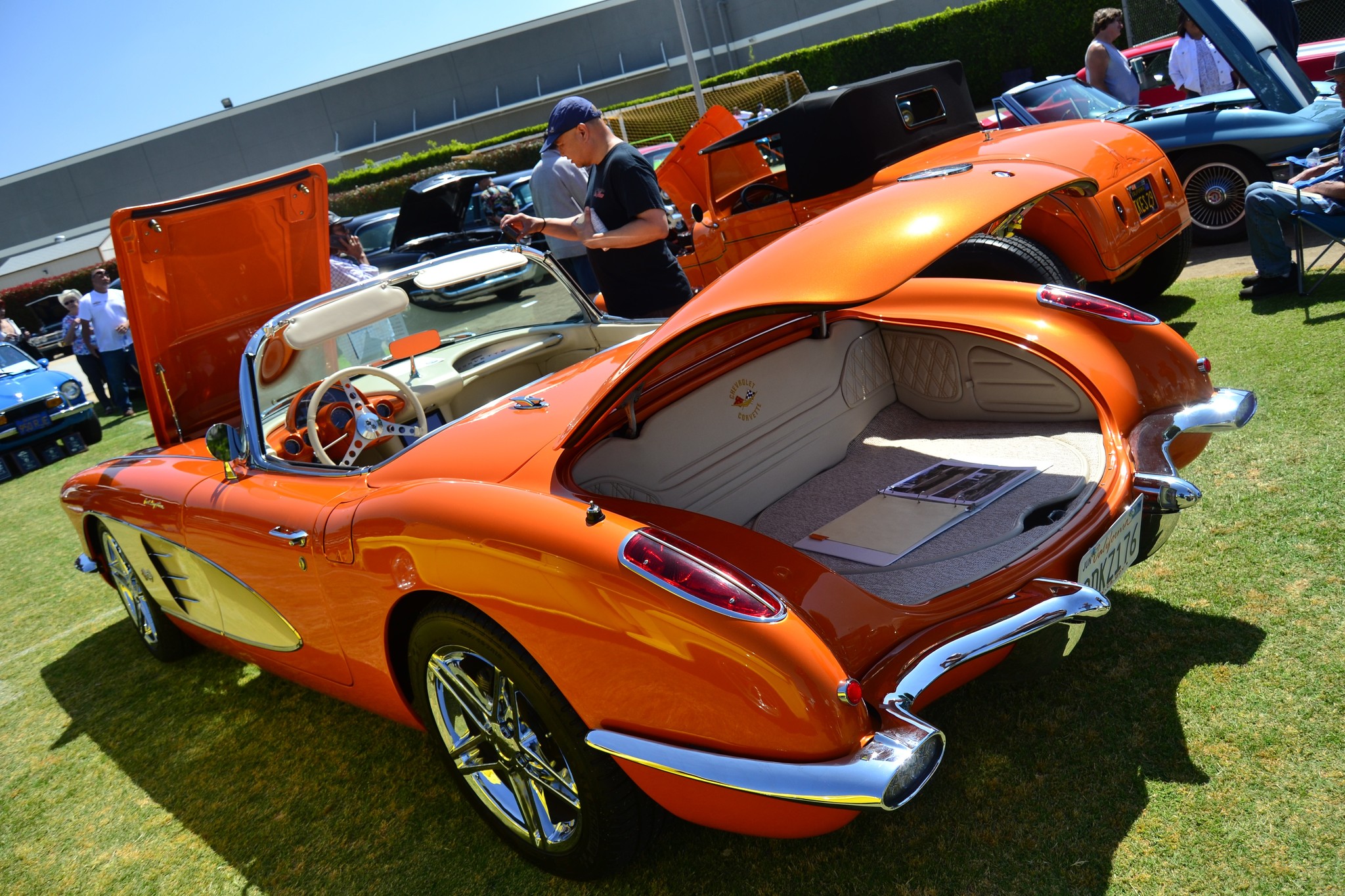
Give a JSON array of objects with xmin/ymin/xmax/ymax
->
[
  {"xmin": 21, "ymin": 294, "xmax": 74, "ymax": 362},
  {"xmin": 0, "ymin": 342, "xmax": 102, "ymax": 484},
  {"xmin": 336, "ymin": 0, "xmax": 1345, "ymax": 308}
]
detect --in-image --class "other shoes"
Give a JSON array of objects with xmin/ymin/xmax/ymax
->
[
  {"xmin": 104, "ymin": 408, "xmax": 115, "ymax": 415},
  {"xmin": 125, "ymin": 409, "xmax": 135, "ymax": 417},
  {"xmin": 1241, "ymin": 261, "xmax": 1299, "ymax": 286},
  {"xmin": 1239, "ymin": 271, "xmax": 1299, "ymax": 300}
]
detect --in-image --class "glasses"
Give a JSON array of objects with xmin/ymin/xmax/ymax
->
[
  {"xmin": 93, "ymin": 270, "xmax": 111, "ymax": 279},
  {"xmin": 64, "ymin": 300, "xmax": 75, "ymax": 306},
  {"xmin": 334, "ymin": 225, "xmax": 347, "ymax": 234},
  {"xmin": 1330, "ymin": 81, "xmax": 1345, "ymax": 91}
]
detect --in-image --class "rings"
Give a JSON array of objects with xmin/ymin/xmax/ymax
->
[{"xmin": 349, "ymin": 240, "xmax": 353, "ymax": 244}]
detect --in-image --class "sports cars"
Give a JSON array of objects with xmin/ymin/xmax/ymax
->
[{"xmin": 54, "ymin": 158, "xmax": 1258, "ymax": 884}]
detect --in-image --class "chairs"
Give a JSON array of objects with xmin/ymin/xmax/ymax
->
[{"xmin": 1286, "ymin": 152, "xmax": 1345, "ymax": 299}]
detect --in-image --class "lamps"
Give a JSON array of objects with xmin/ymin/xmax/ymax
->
[
  {"xmin": 54, "ymin": 235, "xmax": 66, "ymax": 243},
  {"xmin": 221, "ymin": 98, "xmax": 233, "ymax": 109}
]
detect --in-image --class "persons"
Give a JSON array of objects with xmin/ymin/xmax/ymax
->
[
  {"xmin": 726, "ymin": 104, "xmax": 782, "ymax": 166},
  {"xmin": 529, "ymin": 127, "xmax": 601, "ymax": 302},
  {"xmin": 1169, "ymin": 11, "xmax": 1240, "ymax": 99},
  {"xmin": 500, "ymin": 97, "xmax": 693, "ymax": 322},
  {"xmin": 78, "ymin": 268, "xmax": 148, "ymax": 416},
  {"xmin": 1240, "ymin": 51, "xmax": 1345, "ymax": 298},
  {"xmin": 327, "ymin": 210, "xmax": 379, "ymax": 291},
  {"xmin": 0, "ymin": 299, "xmax": 42, "ymax": 360},
  {"xmin": 58, "ymin": 289, "xmax": 120, "ymax": 413},
  {"xmin": 1085, "ymin": 7, "xmax": 1140, "ymax": 107},
  {"xmin": 477, "ymin": 172, "xmax": 520, "ymax": 237}
]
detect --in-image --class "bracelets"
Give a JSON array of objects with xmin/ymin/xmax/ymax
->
[{"xmin": 540, "ymin": 217, "xmax": 546, "ymax": 233}]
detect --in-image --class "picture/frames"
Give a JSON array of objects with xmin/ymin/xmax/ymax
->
[
  {"xmin": 0, "ymin": 454, "xmax": 16, "ymax": 482},
  {"xmin": 8, "ymin": 445, "xmax": 43, "ymax": 475},
  {"xmin": 37, "ymin": 441, "xmax": 65, "ymax": 463},
  {"xmin": 61, "ymin": 431, "xmax": 89, "ymax": 456}
]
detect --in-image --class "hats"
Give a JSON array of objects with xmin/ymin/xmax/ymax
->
[
  {"xmin": 540, "ymin": 96, "xmax": 601, "ymax": 154},
  {"xmin": 328, "ymin": 210, "xmax": 354, "ymax": 226},
  {"xmin": 1325, "ymin": 50, "xmax": 1345, "ymax": 77}
]
